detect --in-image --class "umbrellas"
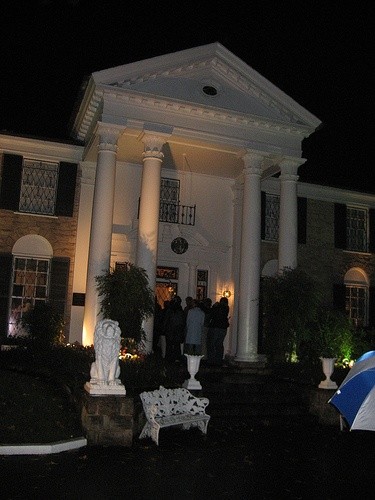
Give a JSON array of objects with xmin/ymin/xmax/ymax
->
[{"xmin": 328, "ymin": 350, "xmax": 375, "ymax": 431}]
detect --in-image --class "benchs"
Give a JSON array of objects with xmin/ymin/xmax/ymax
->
[{"xmin": 137, "ymin": 388, "xmax": 211, "ymax": 446}]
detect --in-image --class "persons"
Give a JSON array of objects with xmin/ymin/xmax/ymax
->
[{"xmin": 155, "ymin": 294, "xmax": 229, "ymax": 361}]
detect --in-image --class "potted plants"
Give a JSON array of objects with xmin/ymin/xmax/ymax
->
[
  {"xmin": 317, "ymin": 313, "xmax": 351, "ymax": 389},
  {"xmin": 181, "ymin": 347, "xmax": 205, "ymax": 389}
]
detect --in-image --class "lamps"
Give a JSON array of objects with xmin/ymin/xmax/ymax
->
[{"xmin": 224, "ymin": 286, "xmax": 231, "ymax": 298}]
